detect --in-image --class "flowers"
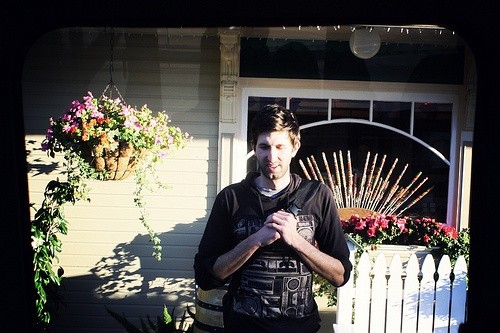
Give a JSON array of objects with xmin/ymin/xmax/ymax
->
[
  {"xmin": 41, "ymin": 91, "xmax": 193, "ymax": 261},
  {"xmin": 314, "ymin": 211, "xmax": 470, "ymax": 262}
]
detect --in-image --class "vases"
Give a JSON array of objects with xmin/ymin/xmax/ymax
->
[{"xmin": 67, "ymin": 140, "xmax": 150, "ymax": 181}]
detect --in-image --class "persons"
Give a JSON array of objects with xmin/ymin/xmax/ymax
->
[{"xmin": 193, "ymin": 104, "xmax": 353, "ymax": 333}]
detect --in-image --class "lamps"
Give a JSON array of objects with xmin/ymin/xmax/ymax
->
[{"xmin": 349, "ymin": 25, "xmax": 381, "ymax": 60}]
